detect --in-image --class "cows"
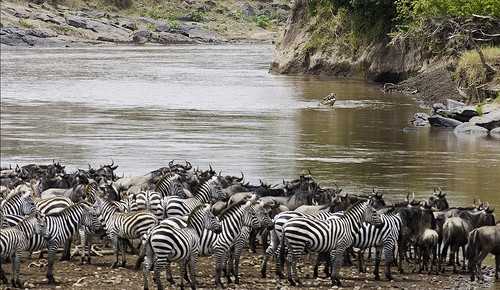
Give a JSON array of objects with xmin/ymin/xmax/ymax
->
[{"xmin": 0, "ymin": 158, "xmax": 500, "ymax": 282}]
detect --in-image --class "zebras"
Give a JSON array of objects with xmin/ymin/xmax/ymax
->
[{"xmin": 0, "ymin": 172, "xmax": 402, "ymax": 290}]
